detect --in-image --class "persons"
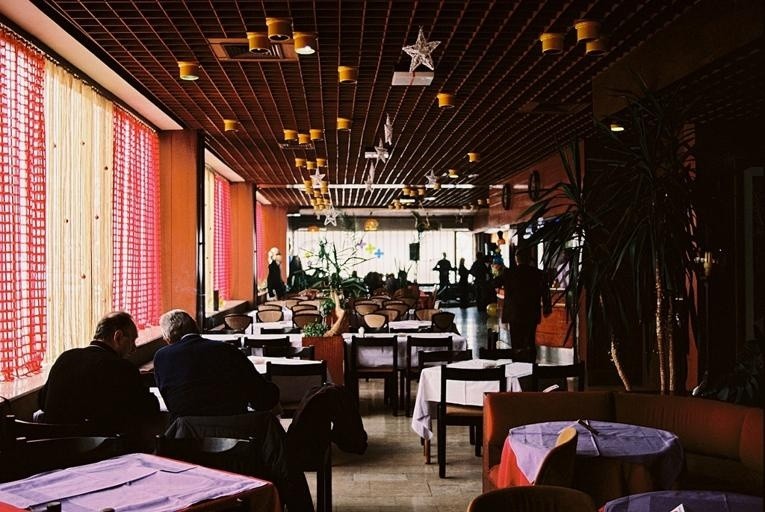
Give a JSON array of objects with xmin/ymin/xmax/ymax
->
[
  {"xmin": 353, "ymin": 253, "xmax": 510, "ymax": 310},
  {"xmin": 37, "ymin": 312, "xmax": 160, "ymax": 436},
  {"xmin": 267, "ymin": 247, "xmax": 286, "ymax": 300},
  {"xmin": 474, "ymin": 246, "xmax": 552, "ymax": 364},
  {"xmin": 153, "ymin": 310, "xmax": 280, "ymax": 425}
]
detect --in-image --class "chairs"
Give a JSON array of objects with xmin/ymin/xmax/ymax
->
[
  {"xmin": 411, "ymin": 329, "xmax": 765, "ymax": 511},
  {"xmin": 0, "ymin": 279, "xmax": 468, "ymax": 511}
]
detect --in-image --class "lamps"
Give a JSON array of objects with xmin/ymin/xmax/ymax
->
[
  {"xmin": 337, "ymin": 117, "xmax": 354, "ymax": 133},
  {"xmin": 223, "ymin": 119, "xmax": 240, "ymax": 133},
  {"xmin": 246, "ymin": 13, "xmax": 319, "ymax": 59},
  {"xmin": 284, "ymin": 128, "xmax": 325, "ymax": 145},
  {"xmin": 468, "ymin": 152, "xmax": 482, "ymax": 164},
  {"xmin": 437, "ymin": 92, "xmax": 457, "ymax": 110},
  {"xmin": 178, "ymin": 61, "xmax": 201, "ymax": 81},
  {"xmin": 337, "ymin": 66, "xmax": 358, "ymax": 85},
  {"xmin": 540, "ymin": 18, "xmax": 612, "ymax": 58},
  {"xmin": 388, "ymin": 168, "xmax": 495, "ymax": 211},
  {"xmin": 295, "ymin": 157, "xmax": 330, "ymax": 213}
]
